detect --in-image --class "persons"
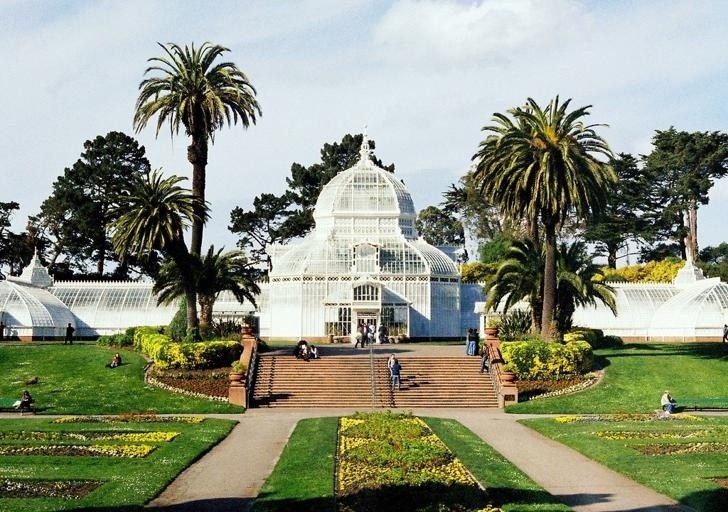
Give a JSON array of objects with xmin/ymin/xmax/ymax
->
[
  {"xmin": 388, "ymin": 354, "xmax": 402, "ymax": 391},
  {"xmin": 661, "ymin": 390, "xmax": 675, "ymax": 414},
  {"xmin": 0, "ymin": 321, "xmax": 6, "ymax": 340},
  {"xmin": 355, "ymin": 323, "xmax": 387, "ymax": 348},
  {"xmin": 722, "ymin": 324, "xmax": 728, "ymax": 343},
  {"xmin": 479, "ymin": 344, "xmax": 489, "ymax": 373},
  {"xmin": 63, "ymin": 323, "xmax": 74, "ymax": 345},
  {"xmin": 295, "ymin": 341, "xmax": 318, "ymax": 362},
  {"xmin": 105, "ymin": 353, "xmax": 121, "ymax": 368},
  {"xmin": 465, "ymin": 328, "xmax": 480, "ymax": 356},
  {"xmin": 15, "ymin": 391, "xmax": 32, "ymax": 416}
]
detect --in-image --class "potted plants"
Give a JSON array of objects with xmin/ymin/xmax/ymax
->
[
  {"xmin": 499, "ymin": 361, "xmax": 519, "ymax": 381},
  {"xmin": 229, "ymin": 361, "xmax": 246, "ymax": 381},
  {"xmin": 241, "ymin": 311, "xmax": 254, "ymax": 333},
  {"xmin": 484, "ymin": 319, "xmax": 498, "ymax": 335}
]
